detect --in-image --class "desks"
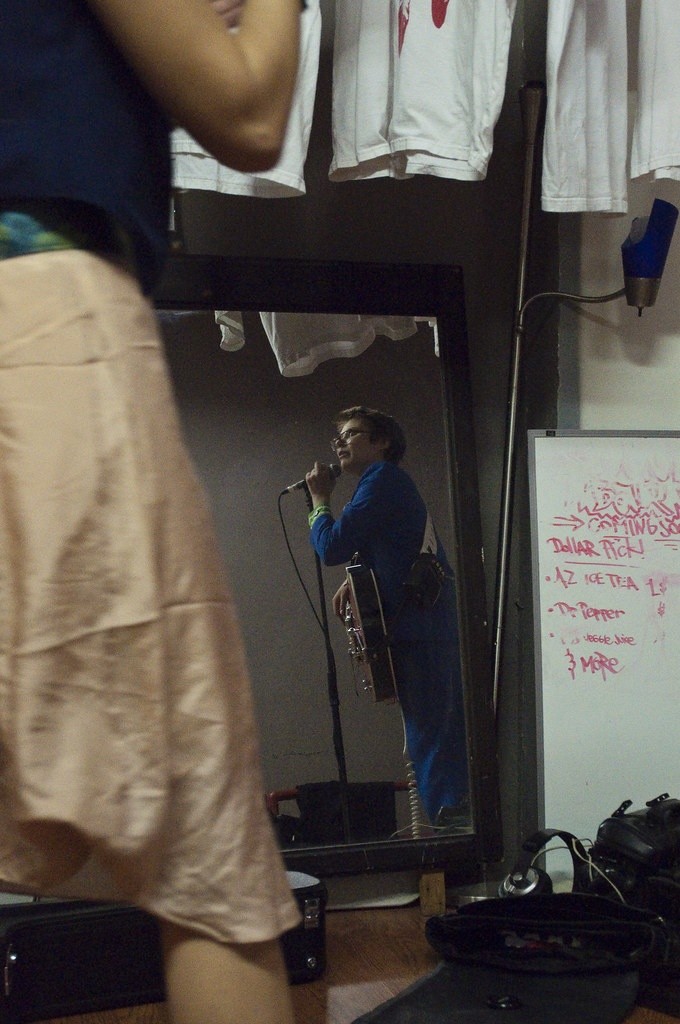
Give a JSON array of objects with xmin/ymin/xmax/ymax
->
[{"xmin": 44, "ymin": 891, "xmax": 461, "ymax": 1023}]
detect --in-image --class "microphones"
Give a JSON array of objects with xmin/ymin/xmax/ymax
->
[{"xmin": 281, "ymin": 463, "xmax": 341, "ymax": 496}]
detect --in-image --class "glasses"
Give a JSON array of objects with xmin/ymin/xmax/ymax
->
[{"xmin": 328, "ymin": 427, "xmax": 378, "ymax": 451}]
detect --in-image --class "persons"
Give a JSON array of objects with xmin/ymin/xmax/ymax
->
[
  {"xmin": 306, "ymin": 407, "xmax": 473, "ymax": 836},
  {"xmin": 0, "ymin": 0, "xmax": 301, "ymax": 1024}
]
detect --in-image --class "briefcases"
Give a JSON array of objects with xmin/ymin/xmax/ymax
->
[{"xmin": 0, "ymin": 864, "xmax": 325, "ymax": 1023}]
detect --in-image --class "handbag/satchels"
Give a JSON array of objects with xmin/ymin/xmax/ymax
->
[{"xmin": 422, "ymin": 891, "xmax": 671, "ymax": 975}]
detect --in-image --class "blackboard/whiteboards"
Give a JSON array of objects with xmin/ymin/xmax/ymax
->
[{"xmin": 523, "ymin": 428, "xmax": 680, "ymax": 892}]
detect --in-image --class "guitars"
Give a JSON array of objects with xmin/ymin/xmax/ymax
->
[{"xmin": 341, "ymin": 564, "xmax": 398, "ymax": 703}]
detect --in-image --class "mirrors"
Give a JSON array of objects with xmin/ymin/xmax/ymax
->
[{"xmin": 142, "ymin": 245, "xmax": 509, "ymax": 887}]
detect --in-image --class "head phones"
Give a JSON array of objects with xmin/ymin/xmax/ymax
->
[{"xmin": 497, "ymin": 828, "xmax": 594, "ymax": 899}]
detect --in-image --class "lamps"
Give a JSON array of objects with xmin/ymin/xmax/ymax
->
[{"xmin": 620, "ymin": 197, "xmax": 680, "ymax": 320}]
